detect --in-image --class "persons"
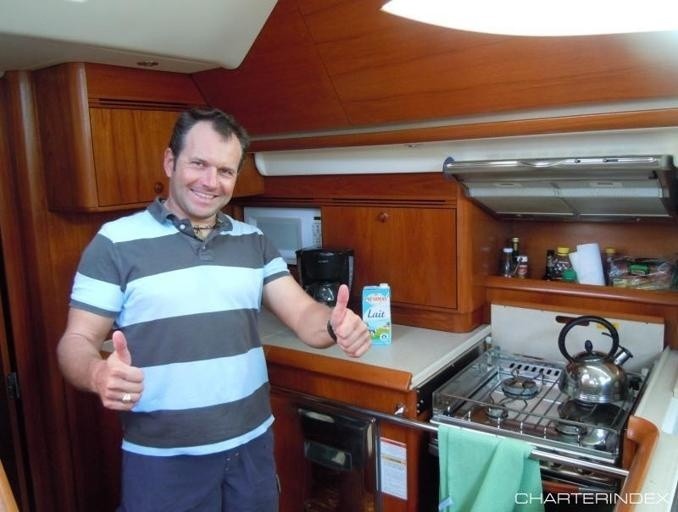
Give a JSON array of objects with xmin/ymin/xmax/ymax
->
[{"xmin": 56, "ymin": 106, "xmax": 371, "ymax": 512}]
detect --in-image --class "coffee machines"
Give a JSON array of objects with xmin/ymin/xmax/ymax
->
[{"xmin": 294, "ymin": 246, "xmax": 357, "ymax": 311}]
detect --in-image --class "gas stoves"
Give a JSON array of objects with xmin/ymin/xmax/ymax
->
[{"xmin": 429, "ymin": 346, "xmax": 646, "ymax": 490}]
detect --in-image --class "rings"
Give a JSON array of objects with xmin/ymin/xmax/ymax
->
[{"xmin": 122, "ymin": 393, "xmax": 131, "ymax": 402}]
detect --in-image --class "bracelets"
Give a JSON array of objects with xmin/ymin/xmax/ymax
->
[{"xmin": 327, "ymin": 318, "xmax": 337, "ymax": 344}]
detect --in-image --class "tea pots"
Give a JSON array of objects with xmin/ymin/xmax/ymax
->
[{"xmin": 555, "ymin": 314, "xmax": 635, "ymax": 404}]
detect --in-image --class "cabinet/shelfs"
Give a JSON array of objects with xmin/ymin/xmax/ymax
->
[{"xmin": 89, "ymin": 94, "xmax": 472, "ymax": 512}]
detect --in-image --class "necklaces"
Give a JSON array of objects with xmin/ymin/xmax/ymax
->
[{"xmin": 193, "ymin": 226, "xmax": 216, "ymax": 230}]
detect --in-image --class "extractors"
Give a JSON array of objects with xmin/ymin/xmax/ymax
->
[{"xmin": 441, "ymin": 151, "xmax": 678, "ymax": 222}]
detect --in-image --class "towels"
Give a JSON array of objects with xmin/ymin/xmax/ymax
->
[{"xmin": 437, "ymin": 425, "xmax": 544, "ymax": 512}]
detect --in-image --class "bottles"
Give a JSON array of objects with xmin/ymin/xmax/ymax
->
[
  {"xmin": 545, "ymin": 245, "xmax": 577, "ymax": 284},
  {"xmin": 499, "ymin": 236, "xmax": 529, "ymax": 279},
  {"xmin": 603, "ymin": 247, "xmax": 616, "ymax": 267}
]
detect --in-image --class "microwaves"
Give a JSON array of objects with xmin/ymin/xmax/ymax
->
[{"xmin": 242, "ymin": 207, "xmax": 322, "ymax": 264}]
